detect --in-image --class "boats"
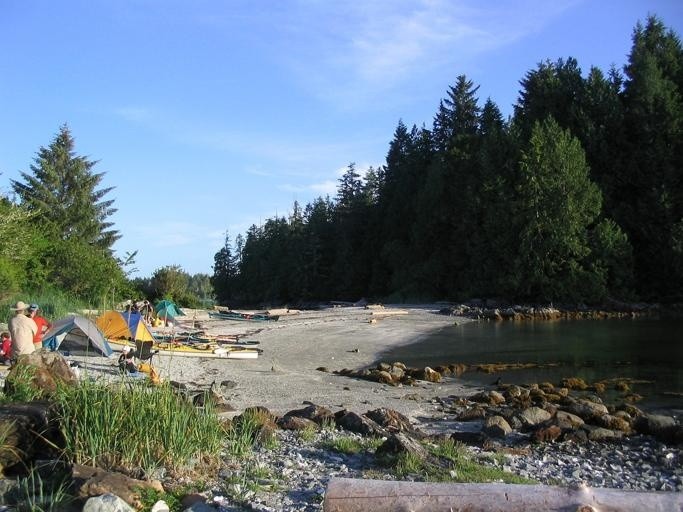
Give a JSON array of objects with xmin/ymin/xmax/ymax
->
[
  {"xmin": 105, "ymin": 332, "xmax": 264, "ymax": 360},
  {"xmin": 330, "ymin": 297, "xmax": 367, "ymax": 305},
  {"xmin": 207, "ymin": 306, "xmax": 298, "ymax": 320}
]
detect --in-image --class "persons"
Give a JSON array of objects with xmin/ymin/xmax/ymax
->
[
  {"xmin": 25, "ymin": 303, "xmax": 53, "ymax": 349},
  {"xmin": 8, "ymin": 301, "xmax": 38, "ymax": 364},
  {"xmin": 132, "ymin": 298, "xmax": 138, "ymax": 309},
  {"xmin": 0, "ymin": 333, "xmax": 11, "ymax": 366},
  {"xmin": 140, "ymin": 300, "xmax": 155, "ymax": 324}
]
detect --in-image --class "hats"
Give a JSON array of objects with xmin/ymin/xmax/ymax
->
[
  {"xmin": 29, "ymin": 304, "xmax": 39, "ymax": 311},
  {"xmin": 9, "ymin": 300, "xmax": 31, "ymax": 312}
]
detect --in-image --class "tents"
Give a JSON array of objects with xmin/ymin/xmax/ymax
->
[
  {"xmin": 96, "ymin": 309, "xmax": 157, "ymax": 343},
  {"xmin": 154, "ymin": 300, "xmax": 186, "ymax": 321},
  {"xmin": 42, "ymin": 314, "xmax": 114, "ymax": 358}
]
